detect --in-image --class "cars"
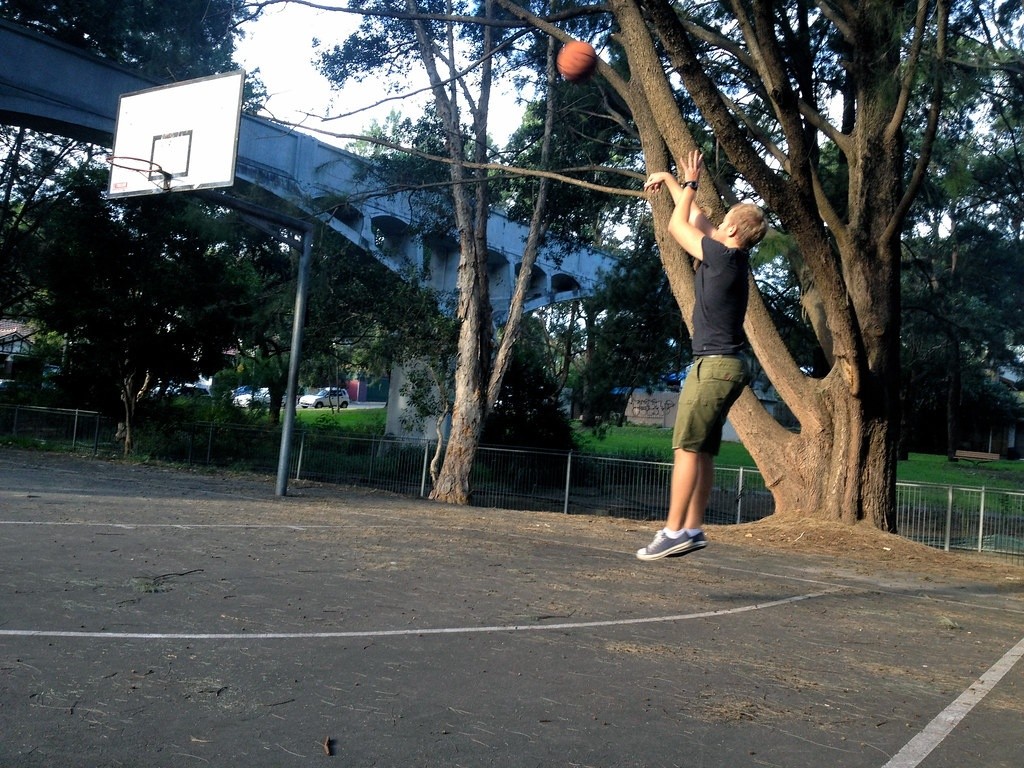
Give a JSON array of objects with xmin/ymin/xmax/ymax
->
[
  {"xmin": 232, "ymin": 387, "xmax": 288, "ymax": 410},
  {"xmin": 151, "ymin": 381, "xmax": 212, "ymax": 401}
]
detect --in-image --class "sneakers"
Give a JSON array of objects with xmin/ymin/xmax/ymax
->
[
  {"xmin": 665, "ymin": 532, "xmax": 709, "ymax": 558},
  {"xmin": 636, "ymin": 529, "xmax": 694, "ymax": 561}
]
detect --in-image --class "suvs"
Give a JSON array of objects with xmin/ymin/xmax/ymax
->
[{"xmin": 298, "ymin": 387, "xmax": 350, "ymax": 410}]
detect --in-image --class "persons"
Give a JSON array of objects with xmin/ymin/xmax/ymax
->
[{"xmin": 636, "ymin": 149, "xmax": 769, "ymax": 562}]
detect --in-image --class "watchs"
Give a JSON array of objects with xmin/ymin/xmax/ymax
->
[{"xmin": 684, "ymin": 181, "xmax": 698, "ymax": 191}]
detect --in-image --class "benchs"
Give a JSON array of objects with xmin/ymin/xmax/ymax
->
[{"xmin": 953, "ymin": 450, "xmax": 1000, "ymax": 466}]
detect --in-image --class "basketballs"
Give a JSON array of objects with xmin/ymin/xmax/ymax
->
[{"xmin": 557, "ymin": 40, "xmax": 597, "ymax": 80}]
